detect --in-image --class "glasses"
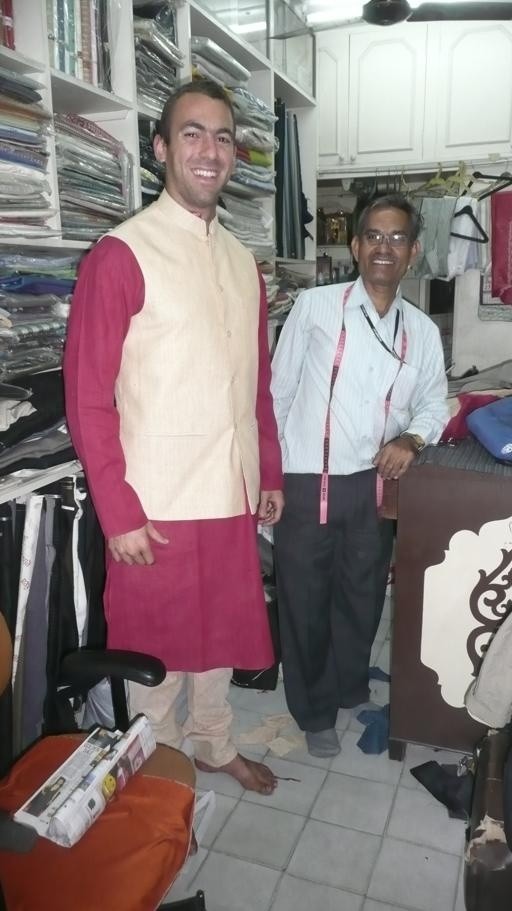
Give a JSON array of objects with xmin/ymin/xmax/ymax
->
[{"xmin": 364, "ymin": 231, "xmax": 410, "ymax": 248}]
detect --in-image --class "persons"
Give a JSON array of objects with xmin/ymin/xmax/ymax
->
[
  {"xmin": 269, "ymin": 189, "xmax": 450, "ymax": 759},
  {"xmin": 65, "ymin": 74, "xmax": 287, "ymax": 853}
]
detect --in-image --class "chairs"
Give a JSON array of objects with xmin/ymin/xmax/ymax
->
[{"xmin": 1, "ymin": 647, "xmax": 210, "ymax": 911}]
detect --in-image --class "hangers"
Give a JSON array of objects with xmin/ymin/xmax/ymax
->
[{"xmin": 334, "ymin": 166, "xmax": 510, "ymax": 292}]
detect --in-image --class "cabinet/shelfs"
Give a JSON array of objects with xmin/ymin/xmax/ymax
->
[
  {"xmin": 285, "ymin": 12, "xmax": 512, "ymax": 169},
  {"xmin": 3, "ymin": 2, "xmax": 322, "ymax": 510}
]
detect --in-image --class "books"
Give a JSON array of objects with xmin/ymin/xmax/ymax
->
[{"xmin": 14, "ymin": 713, "xmax": 164, "ymax": 847}]
